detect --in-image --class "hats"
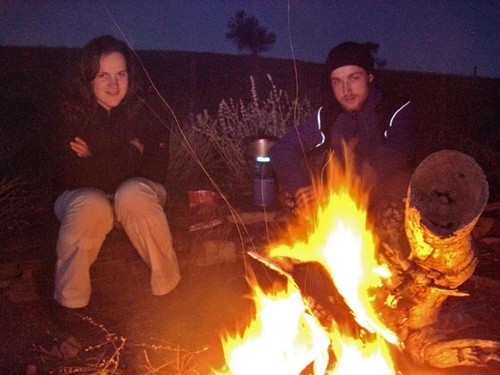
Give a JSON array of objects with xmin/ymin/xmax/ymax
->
[{"xmin": 326, "ymin": 41, "xmax": 374, "ymax": 72}]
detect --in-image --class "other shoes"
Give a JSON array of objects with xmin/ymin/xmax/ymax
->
[{"xmin": 45, "ymin": 296, "xmax": 90, "ymax": 329}]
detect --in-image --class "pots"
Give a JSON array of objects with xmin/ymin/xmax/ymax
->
[{"xmin": 244, "ymin": 129, "xmax": 280, "ymax": 156}]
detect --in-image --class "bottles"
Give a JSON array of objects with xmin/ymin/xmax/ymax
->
[{"xmin": 253, "ymin": 156, "xmax": 276, "ymax": 209}]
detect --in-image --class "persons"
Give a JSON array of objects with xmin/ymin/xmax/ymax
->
[
  {"xmin": 45, "ymin": 35, "xmax": 185, "ymax": 323},
  {"xmin": 266, "ymin": 41, "xmax": 418, "ymax": 208}
]
